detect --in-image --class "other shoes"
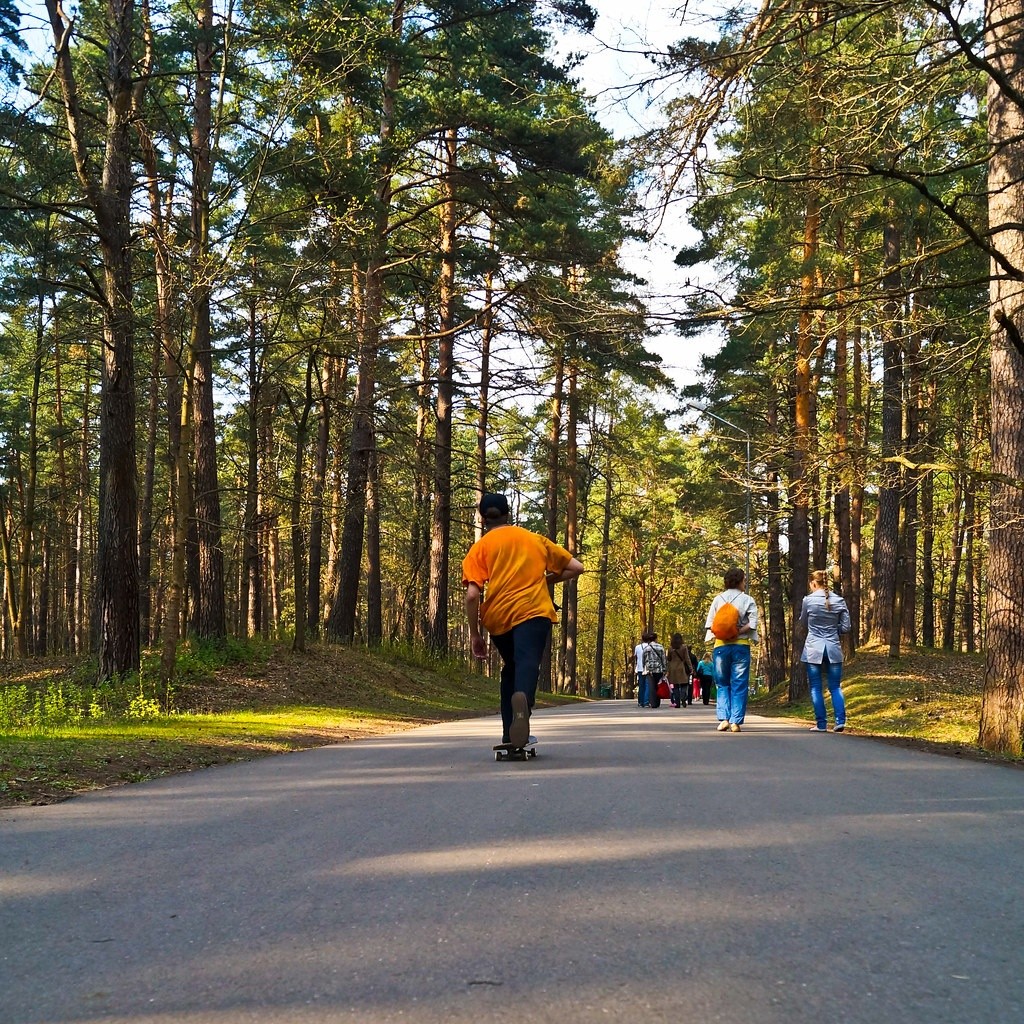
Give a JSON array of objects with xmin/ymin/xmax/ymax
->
[
  {"xmin": 704, "ymin": 702, "xmax": 709, "ymax": 705},
  {"xmin": 644, "ymin": 704, "xmax": 650, "ymax": 708},
  {"xmin": 810, "ymin": 726, "xmax": 827, "ymax": 732},
  {"xmin": 688, "ymin": 702, "xmax": 692, "ymax": 705},
  {"xmin": 675, "ymin": 704, "xmax": 680, "ymax": 708},
  {"xmin": 510, "ymin": 692, "xmax": 530, "ymax": 748},
  {"xmin": 717, "ymin": 721, "xmax": 729, "ymax": 731},
  {"xmin": 834, "ymin": 723, "xmax": 844, "ymax": 732},
  {"xmin": 682, "ymin": 701, "xmax": 686, "ymax": 708},
  {"xmin": 730, "ymin": 723, "xmax": 740, "ymax": 732},
  {"xmin": 638, "ymin": 704, "xmax": 643, "ymax": 707},
  {"xmin": 502, "ymin": 736, "xmax": 512, "ymax": 745}
]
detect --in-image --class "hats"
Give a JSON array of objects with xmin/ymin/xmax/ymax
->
[{"xmin": 479, "ymin": 494, "xmax": 508, "ymax": 518}]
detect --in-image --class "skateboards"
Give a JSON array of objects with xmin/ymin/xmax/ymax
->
[{"xmin": 493, "ymin": 735, "xmax": 538, "ymax": 761}]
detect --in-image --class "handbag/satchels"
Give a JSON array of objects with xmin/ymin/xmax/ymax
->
[
  {"xmin": 683, "ymin": 661, "xmax": 691, "ymax": 675},
  {"xmin": 697, "ymin": 666, "xmax": 703, "ymax": 678},
  {"xmin": 656, "ymin": 680, "xmax": 671, "ymax": 699}
]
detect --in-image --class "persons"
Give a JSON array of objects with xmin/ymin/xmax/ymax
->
[
  {"xmin": 665, "ymin": 634, "xmax": 693, "ymax": 708},
  {"xmin": 705, "ymin": 568, "xmax": 758, "ymax": 731},
  {"xmin": 799, "ymin": 571, "xmax": 850, "ymax": 731},
  {"xmin": 686, "ymin": 644, "xmax": 697, "ymax": 705},
  {"xmin": 693, "ymin": 678, "xmax": 701, "ymax": 702},
  {"xmin": 462, "ymin": 493, "xmax": 584, "ymax": 749},
  {"xmin": 697, "ymin": 654, "xmax": 714, "ymax": 705},
  {"xmin": 634, "ymin": 634, "xmax": 650, "ymax": 708},
  {"xmin": 642, "ymin": 633, "xmax": 666, "ymax": 708}
]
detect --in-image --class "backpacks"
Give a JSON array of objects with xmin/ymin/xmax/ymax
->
[{"xmin": 711, "ymin": 592, "xmax": 742, "ymax": 642}]
